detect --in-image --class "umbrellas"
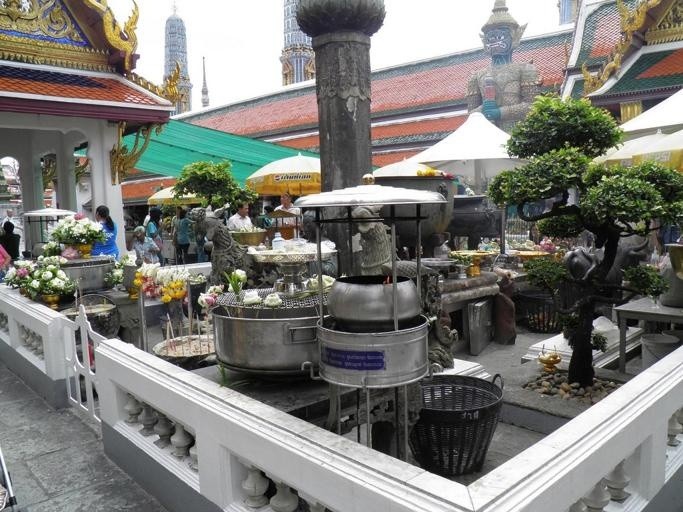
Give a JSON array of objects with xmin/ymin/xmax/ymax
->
[
  {"xmin": 402, "ymin": 112, "xmax": 539, "ymax": 163},
  {"xmin": 596, "ymin": 86, "xmax": 683, "ymax": 137},
  {"xmin": 592, "ymin": 130, "xmax": 683, "ymax": 163},
  {"xmin": 145, "ymin": 185, "xmax": 204, "ymax": 207},
  {"xmin": 244, "ymin": 152, "xmax": 321, "ymax": 197},
  {"xmin": 373, "ymin": 161, "xmax": 440, "ymax": 176}
]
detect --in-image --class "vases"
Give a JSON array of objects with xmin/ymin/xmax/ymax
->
[
  {"xmin": 72, "ymin": 244, "xmax": 93, "ymax": 259},
  {"xmin": 42, "ymin": 295, "xmax": 61, "ymax": 309}
]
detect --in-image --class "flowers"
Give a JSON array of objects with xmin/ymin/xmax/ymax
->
[
  {"xmin": 133, "ymin": 260, "xmax": 348, "ymax": 308},
  {"xmin": 50, "ymin": 212, "xmax": 114, "ymax": 246},
  {"xmin": 2, "ymin": 255, "xmax": 82, "ymax": 301}
]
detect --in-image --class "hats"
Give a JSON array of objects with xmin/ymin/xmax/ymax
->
[{"xmin": 150, "ymin": 208, "xmax": 163, "ymax": 219}]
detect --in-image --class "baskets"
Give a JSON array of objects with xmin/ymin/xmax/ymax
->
[
  {"xmin": 516, "ymin": 288, "xmax": 562, "ymax": 335},
  {"xmin": 409, "ymin": 373, "xmax": 505, "ymax": 476}
]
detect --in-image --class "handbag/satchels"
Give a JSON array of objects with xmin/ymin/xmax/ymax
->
[{"xmin": 144, "ymin": 235, "xmax": 161, "ymax": 252}]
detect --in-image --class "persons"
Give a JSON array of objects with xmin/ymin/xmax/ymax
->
[
  {"xmin": 529, "ymin": 224, "xmax": 542, "ymax": 244},
  {"xmin": 262, "ymin": 196, "xmax": 303, "ymax": 245},
  {"xmin": 466, "ymin": 0, "xmax": 545, "ymax": 133},
  {"xmin": 657, "ymin": 217, "xmax": 680, "ymax": 250},
  {"xmin": 1, "ymin": 209, "xmax": 25, "ymax": 272},
  {"xmin": 645, "ymin": 236, "xmax": 674, "ymax": 275},
  {"xmin": 130, "ymin": 198, "xmax": 253, "ymax": 266},
  {"xmin": 91, "ymin": 205, "xmax": 118, "ymax": 261}
]
